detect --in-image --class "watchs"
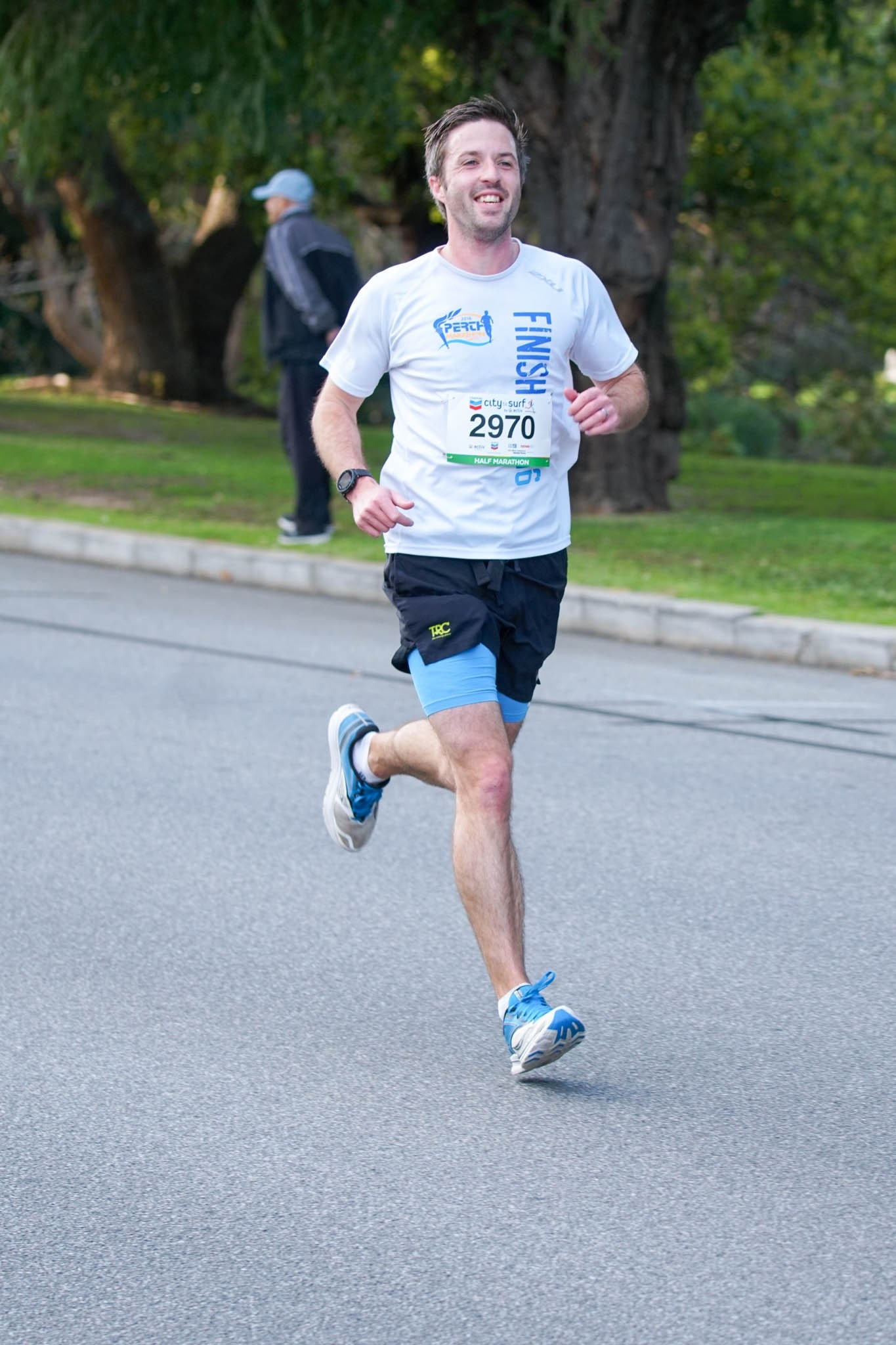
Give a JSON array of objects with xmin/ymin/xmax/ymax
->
[{"xmin": 336, "ymin": 467, "xmax": 373, "ymax": 505}]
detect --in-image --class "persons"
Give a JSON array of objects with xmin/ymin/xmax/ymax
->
[
  {"xmin": 252, "ymin": 169, "xmax": 360, "ymax": 547},
  {"xmin": 310, "ymin": 95, "xmax": 646, "ymax": 1083}
]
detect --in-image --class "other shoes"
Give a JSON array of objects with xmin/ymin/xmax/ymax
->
[{"xmin": 277, "ymin": 511, "xmax": 338, "ymax": 548}]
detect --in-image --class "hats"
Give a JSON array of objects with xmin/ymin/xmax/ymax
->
[{"xmin": 252, "ymin": 167, "xmax": 316, "ymax": 204}]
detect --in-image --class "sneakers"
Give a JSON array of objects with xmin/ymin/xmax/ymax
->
[
  {"xmin": 321, "ymin": 702, "xmax": 391, "ymax": 854},
  {"xmin": 502, "ymin": 969, "xmax": 588, "ymax": 1076}
]
